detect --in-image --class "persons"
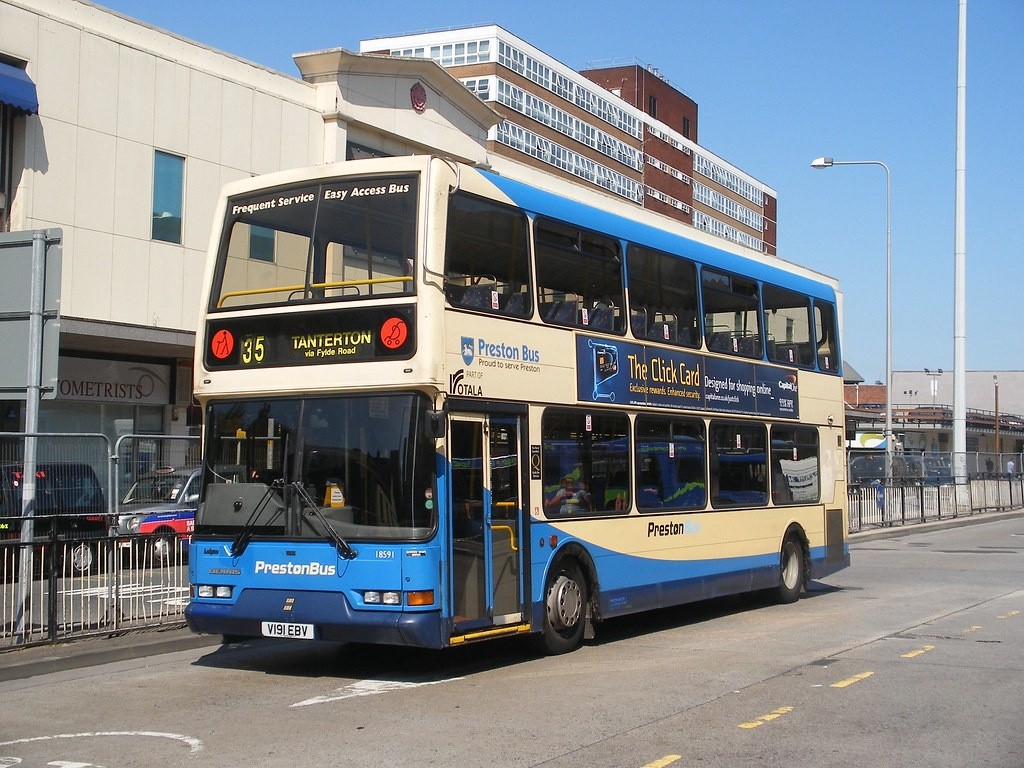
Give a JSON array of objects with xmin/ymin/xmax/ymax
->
[
  {"xmin": 985, "ymin": 457, "xmax": 995, "ymax": 477},
  {"xmin": 1006, "ymin": 458, "xmax": 1015, "ymax": 479},
  {"xmin": 552, "ymin": 472, "xmax": 595, "ymax": 515},
  {"xmin": 929, "ymin": 438, "xmax": 940, "ymax": 467},
  {"xmin": 262, "ymin": 428, "xmax": 328, "ymax": 509}
]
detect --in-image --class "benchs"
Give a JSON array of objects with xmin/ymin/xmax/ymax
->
[{"xmin": 443, "ymin": 274, "xmax": 838, "ymax": 374}]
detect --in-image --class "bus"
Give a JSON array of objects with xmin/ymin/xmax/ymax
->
[{"xmin": 183, "ymin": 154, "xmax": 853, "ymax": 658}]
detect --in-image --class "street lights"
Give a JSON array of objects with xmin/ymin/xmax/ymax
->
[{"xmin": 810, "ymin": 155, "xmax": 894, "ymax": 489}]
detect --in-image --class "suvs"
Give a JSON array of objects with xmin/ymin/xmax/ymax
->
[{"xmin": 100, "ymin": 465, "xmax": 248, "ymax": 562}]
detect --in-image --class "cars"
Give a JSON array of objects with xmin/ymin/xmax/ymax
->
[
  {"xmin": 845, "ymin": 401, "xmax": 1024, "ymax": 430},
  {"xmin": 846, "ymin": 451, "xmax": 955, "ymax": 488}
]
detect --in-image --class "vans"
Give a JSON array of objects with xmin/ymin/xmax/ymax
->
[{"xmin": 0, "ymin": 461, "xmax": 110, "ymax": 574}]
